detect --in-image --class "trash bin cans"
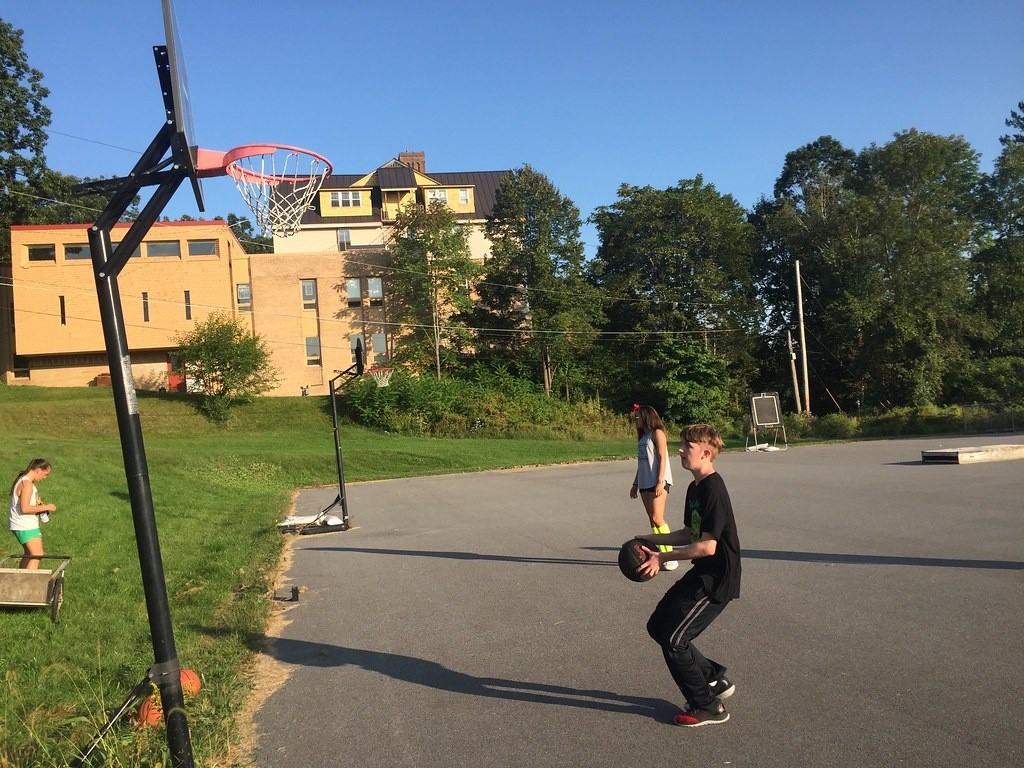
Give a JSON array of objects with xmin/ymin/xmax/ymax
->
[{"xmin": 95, "ymin": 372, "xmax": 112, "ymax": 386}]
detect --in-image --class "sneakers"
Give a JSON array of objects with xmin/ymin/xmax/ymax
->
[
  {"xmin": 674, "ymin": 700, "xmax": 730, "ymax": 727},
  {"xmin": 680, "ymin": 676, "xmax": 736, "ymax": 712}
]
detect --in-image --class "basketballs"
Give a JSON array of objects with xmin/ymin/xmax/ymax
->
[
  {"xmin": 617, "ymin": 538, "xmax": 661, "ymax": 582},
  {"xmin": 138, "ymin": 696, "xmax": 163, "ymax": 726},
  {"xmin": 180, "ymin": 670, "xmax": 199, "ymax": 695}
]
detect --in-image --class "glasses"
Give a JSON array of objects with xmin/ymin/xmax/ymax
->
[{"xmin": 635, "ymin": 416, "xmax": 642, "ymax": 420}]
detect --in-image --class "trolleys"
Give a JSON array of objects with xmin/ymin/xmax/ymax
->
[{"xmin": 0, "ymin": 555, "xmax": 73, "ymax": 623}]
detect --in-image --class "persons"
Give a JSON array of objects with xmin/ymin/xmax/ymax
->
[
  {"xmin": 629, "ymin": 404, "xmax": 679, "ymax": 571},
  {"xmin": 621, "ymin": 423, "xmax": 742, "ymax": 726},
  {"xmin": 8, "ymin": 459, "xmax": 56, "ymax": 569}
]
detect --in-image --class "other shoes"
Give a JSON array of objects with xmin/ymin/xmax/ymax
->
[{"xmin": 662, "ymin": 560, "xmax": 679, "ymax": 570}]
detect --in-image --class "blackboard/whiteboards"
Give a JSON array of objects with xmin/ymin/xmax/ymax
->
[{"xmin": 749, "ymin": 392, "xmax": 784, "ymax": 430}]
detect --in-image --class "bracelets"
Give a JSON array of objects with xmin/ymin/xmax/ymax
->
[
  {"xmin": 632, "ymin": 481, "xmax": 638, "ymax": 486},
  {"xmin": 657, "ymin": 480, "xmax": 663, "ymax": 484}
]
